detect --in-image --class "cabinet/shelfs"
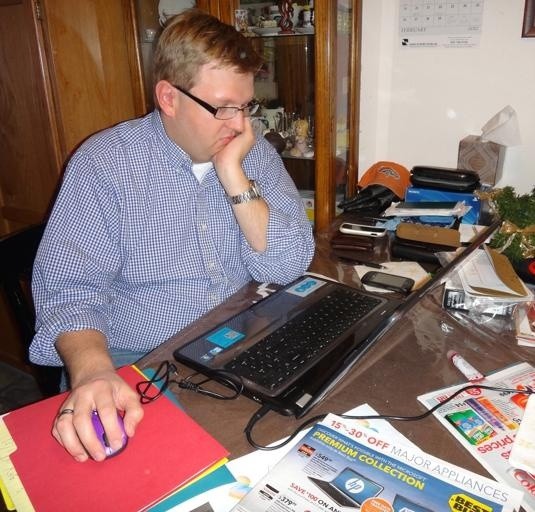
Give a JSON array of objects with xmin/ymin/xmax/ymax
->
[{"xmin": 124, "ymin": 0, "xmax": 364, "ymax": 244}]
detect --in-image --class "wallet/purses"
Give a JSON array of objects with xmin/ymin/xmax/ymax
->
[{"xmin": 328, "ymin": 234, "xmax": 376, "ymax": 253}]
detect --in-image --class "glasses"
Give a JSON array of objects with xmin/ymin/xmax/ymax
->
[{"xmin": 173, "ymin": 84, "xmax": 260, "ymax": 120}]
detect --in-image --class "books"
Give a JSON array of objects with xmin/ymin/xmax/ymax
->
[
  {"xmin": 445, "ymin": 226, "xmax": 535, "ymax": 348},
  {"xmin": 383, "ymin": 202, "xmax": 472, "ymax": 217}
]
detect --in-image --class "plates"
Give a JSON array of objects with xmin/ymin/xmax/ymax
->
[
  {"xmin": 296, "ymin": 27, "xmax": 314, "ymax": 34},
  {"xmin": 253, "ymin": 27, "xmax": 281, "ymax": 37}
]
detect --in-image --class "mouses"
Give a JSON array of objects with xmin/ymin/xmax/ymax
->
[{"xmin": 85, "ymin": 409, "xmax": 128, "ymax": 461}]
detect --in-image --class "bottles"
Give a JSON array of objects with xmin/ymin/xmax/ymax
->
[{"xmin": 303, "ymin": 5, "xmax": 311, "ymax": 26}]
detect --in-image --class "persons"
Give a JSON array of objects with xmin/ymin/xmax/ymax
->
[{"xmin": 28, "ymin": 12, "xmax": 316, "ymax": 462}]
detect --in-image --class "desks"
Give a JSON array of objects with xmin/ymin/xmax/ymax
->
[{"xmin": 133, "ymin": 210, "xmax": 535, "ymax": 511}]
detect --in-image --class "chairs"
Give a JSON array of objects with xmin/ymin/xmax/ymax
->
[{"xmin": 2, "ymin": 222, "xmax": 67, "ymax": 397}]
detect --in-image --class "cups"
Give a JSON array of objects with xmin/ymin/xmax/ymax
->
[{"xmin": 273, "ymin": 111, "xmax": 313, "ymax": 140}]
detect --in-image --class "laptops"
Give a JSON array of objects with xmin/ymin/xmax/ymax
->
[
  {"xmin": 173, "ymin": 218, "xmax": 503, "ymax": 419},
  {"xmin": 307, "ymin": 467, "xmax": 385, "ymax": 508}
]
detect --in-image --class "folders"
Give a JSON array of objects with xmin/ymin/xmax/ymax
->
[{"xmin": 0, "ymin": 363, "xmax": 239, "ymax": 512}]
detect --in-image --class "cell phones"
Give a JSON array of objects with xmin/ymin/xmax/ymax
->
[
  {"xmin": 339, "ymin": 222, "xmax": 387, "ymax": 238},
  {"xmin": 361, "ymin": 270, "xmax": 414, "ymax": 294}
]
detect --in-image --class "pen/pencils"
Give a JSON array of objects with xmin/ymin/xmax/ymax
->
[{"xmin": 338, "ymin": 257, "xmax": 387, "ymax": 269}]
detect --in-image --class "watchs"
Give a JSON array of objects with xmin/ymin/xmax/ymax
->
[{"xmin": 223, "ymin": 179, "xmax": 262, "ymax": 205}]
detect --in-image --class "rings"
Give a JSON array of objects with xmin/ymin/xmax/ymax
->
[{"xmin": 58, "ymin": 410, "xmax": 75, "ymax": 416}]
